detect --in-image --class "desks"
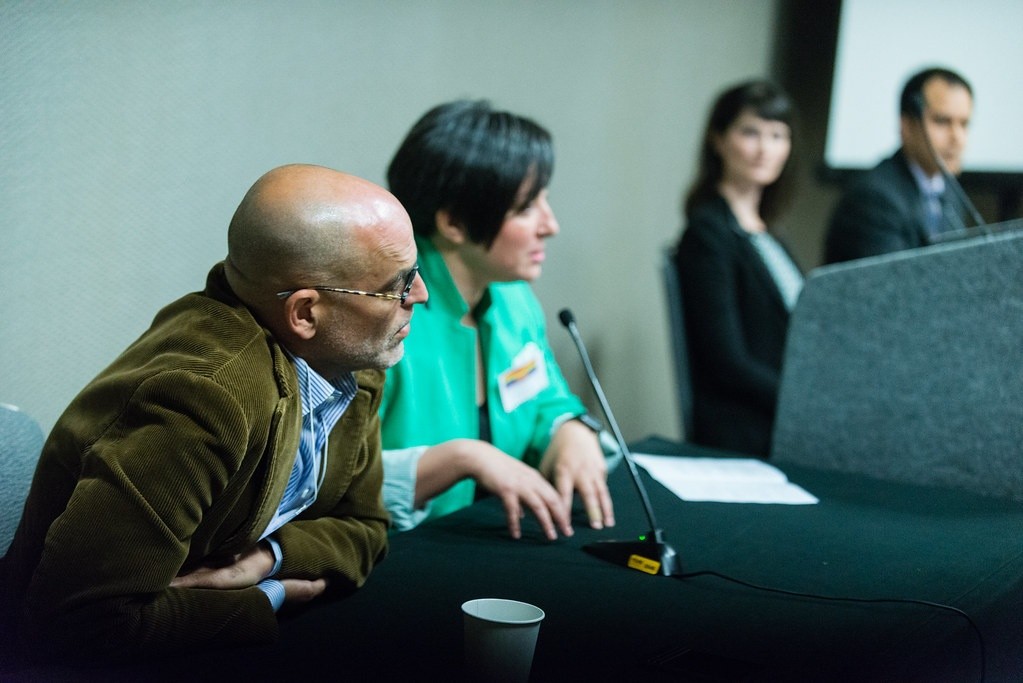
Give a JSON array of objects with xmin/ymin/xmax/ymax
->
[{"xmin": 0, "ymin": 436, "xmax": 1023, "ymax": 683}]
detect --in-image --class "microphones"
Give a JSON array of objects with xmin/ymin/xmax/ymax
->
[
  {"xmin": 559, "ymin": 308, "xmax": 685, "ymax": 577},
  {"xmin": 907, "ymin": 94, "xmax": 987, "ymax": 229}
]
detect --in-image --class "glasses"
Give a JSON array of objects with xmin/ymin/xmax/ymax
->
[{"xmin": 276, "ymin": 266, "xmax": 419, "ymax": 305}]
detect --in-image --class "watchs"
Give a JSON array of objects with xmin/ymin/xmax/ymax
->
[{"xmin": 566, "ymin": 412, "xmax": 603, "ymax": 437}]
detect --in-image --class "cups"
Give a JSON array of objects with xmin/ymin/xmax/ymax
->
[{"xmin": 461, "ymin": 598, "xmax": 545, "ymax": 683}]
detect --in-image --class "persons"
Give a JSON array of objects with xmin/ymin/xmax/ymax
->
[
  {"xmin": 0, "ymin": 164, "xmax": 429, "ymax": 669},
  {"xmin": 821, "ymin": 68, "xmax": 980, "ymax": 267},
  {"xmin": 378, "ymin": 97, "xmax": 625, "ymax": 540},
  {"xmin": 675, "ymin": 80, "xmax": 809, "ymax": 461}
]
